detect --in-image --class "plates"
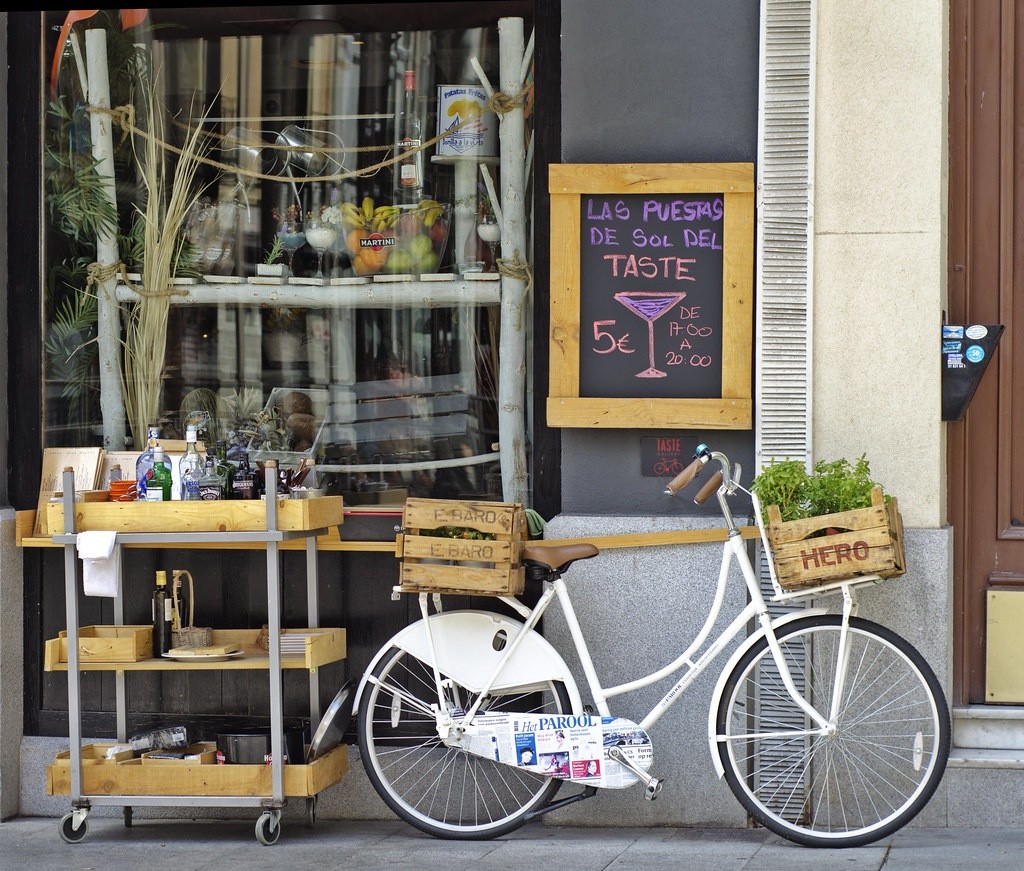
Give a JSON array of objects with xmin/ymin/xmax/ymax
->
[
  {"xmin": 160, "ymin": 649, "xmax": 245, "ymax": 662},
  {"xmin": 268, "ymin": 633, "xmax": 322, "ymax": 654}
]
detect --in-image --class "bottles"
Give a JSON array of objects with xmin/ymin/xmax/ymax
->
[
  {"xmin": 152, "ymin": 571, "xmax": 173, "ymax": 658},
  {"xmin": 178, "ymin": 424, "xmax": 205, "ymax": 500},
  {"xmin": 197, "ymin": 447, "xmax": 224, "ymax": 501},
  {"xmin": 215, "ymin": 440, "xmax": 236, "ymax": 501},
  {"xmin": 144, "ymin": 446, "xmax": 172, "ymax": 502},
  {"xmin": 136, "ymin": 424, "xmax": 172, "ymax": 501},
  {"xmin": 170, "ymin": 570, "xmax": 187, "ymax": 649},
  {"xmin": 390, "ymin": 70, "xmax": 423, "ymax": 198},
  {"xmin": 233, "ymin": 453, "xmax": 261, "ymax": 499}
]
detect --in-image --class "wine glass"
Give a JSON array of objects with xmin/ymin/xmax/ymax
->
[
  {"xmin": 277, "ymin": 222, "xmax": 307, "ymax": 279},
  {"xmin": 327, "ymin": 223, "xmax": 346, "ymax": 278},
  {"xmin": 473, "ymin": 214, "xmax": 502, "ymax": 272},
  {"xmin": 305, "ymin": 220, "xmax": 337, "ymax": 279}
]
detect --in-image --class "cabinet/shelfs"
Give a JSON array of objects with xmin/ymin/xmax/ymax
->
[{"xmin": 16, "ymin": 494, "xmax": 347, "ymax": 845}]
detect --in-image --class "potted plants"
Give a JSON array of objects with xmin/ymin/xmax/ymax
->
[
  {"xmin": 477, "ymin": 183, "xmax": 501, "ymax": 273},
  {"xmin": 254, "ymin": 235, "xmax": 289, "ymax": 279},
  {"xmin": 749, "ymin": 453, "xmax": 907, "ymax": 591},
  {"xmin": 399, "ymin": 497, "xmax": 527, "ymax": 596}
]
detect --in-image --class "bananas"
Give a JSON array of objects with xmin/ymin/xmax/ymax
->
[{"xmin": 340, "ymin": 197, "xmax": 444, "ymax": 232}]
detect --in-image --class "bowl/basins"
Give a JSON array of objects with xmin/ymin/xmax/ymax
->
[
  {"xmin": 110, "ymin": 479, "xmax": 137, "ymax": 502},
  {"xmin": 332, "ymin": 203, "xmax": 453, "ymax": 277}
]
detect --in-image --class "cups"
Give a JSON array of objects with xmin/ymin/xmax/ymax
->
[
  {"xmin": 274, "ymin": 125, "xmax": 329, "ymax": 175},
  {"xmin": 222, "ymin": 127, "xmax": 281, "ymax": 175},
  {"xmin": 290, "ymin": 488, "xmax": 325, "ymax": 499},
  {"xmin": 260, "ymin": 494, "xmax": 290, "ymax": 501}
]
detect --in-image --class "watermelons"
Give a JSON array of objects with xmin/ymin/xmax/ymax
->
[{"xmin": 178, "ymin": 387, "xmax": 219, "ymax": 443}]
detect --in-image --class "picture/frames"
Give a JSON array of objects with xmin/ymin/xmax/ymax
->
[{"xmin": 547, "ymin": 162, "xmax": 754, "ymax": 430}]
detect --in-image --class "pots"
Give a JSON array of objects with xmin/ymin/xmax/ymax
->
[{"xmin": 214, "ymin": 720, "xmax": 310, "ymax": 764}]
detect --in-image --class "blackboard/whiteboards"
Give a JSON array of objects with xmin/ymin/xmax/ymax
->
[{"xmin": 544, "ymin": 161, "xmax": 755, "ymax": 433}]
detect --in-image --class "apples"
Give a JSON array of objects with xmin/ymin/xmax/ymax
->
[{"xmin": 346, "ymin": 213, "xmax": 448, "ymax": 275}]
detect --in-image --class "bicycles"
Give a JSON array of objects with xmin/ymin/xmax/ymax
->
[{"xmin": 350, "ymin": 443, "xmax": 953, "ymax": 848}]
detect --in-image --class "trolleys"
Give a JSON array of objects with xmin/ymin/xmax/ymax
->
[{"xmin": 57, "ymin": 458, "xmax": 320, "ymax": 846}]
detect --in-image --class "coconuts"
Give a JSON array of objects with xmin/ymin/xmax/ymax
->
[{"xmin": 278, "ymin": 391, "xmax": 318, "ymax": 451}]
